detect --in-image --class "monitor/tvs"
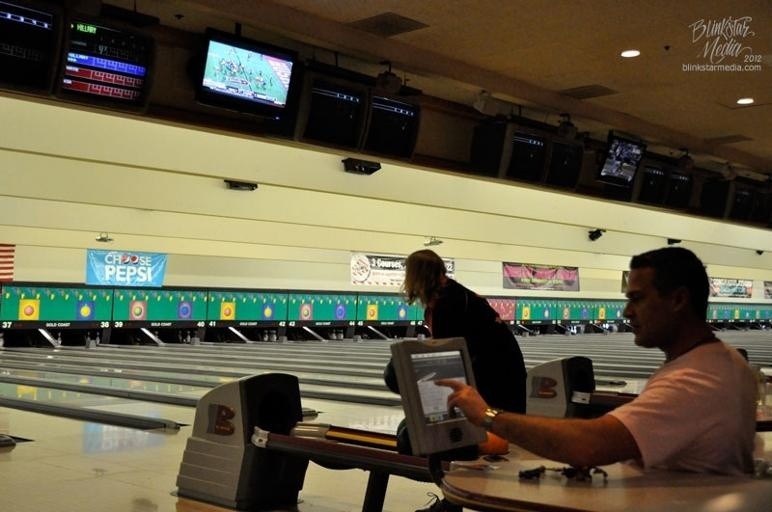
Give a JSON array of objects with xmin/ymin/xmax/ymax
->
[
  {"xmin": 0, "ymin": 0, "xmax": 422, "ymax": 162},
  {"xmin": 467, "ymin": 120, "xmax": 772, "ymax": 231},
  {"xmin": 390, "ymin": 336, "xmax": 487, "ymax": 457}
]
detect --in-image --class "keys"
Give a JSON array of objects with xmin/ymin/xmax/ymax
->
[{"xmin": 519, "ymin": 464, "xmax": 546, "ymax": 477}]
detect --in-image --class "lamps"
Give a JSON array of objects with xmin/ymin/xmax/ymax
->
[
  {"xmin": 373, "ymin": 58, "xmax": 402, "ymax": 99},
  {"xmin": 556, "ymin": 111, "xmax": 577, "ymax": 140},
  {"xmin": 675, "ymin": 146, "xmax": 694, "ymax": 173}
]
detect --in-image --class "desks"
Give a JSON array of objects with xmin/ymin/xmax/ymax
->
[{"xmin": 440, "ymin": 460, "xmax": 772, "ymax": 512}]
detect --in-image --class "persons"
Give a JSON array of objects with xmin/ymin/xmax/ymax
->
[
  {"xmin": 385, "ymin": 249, "xmax": 528, "ymax": 511},
  {"xmin": 434, "ymin": 246, "xmax": 760, "ymax": 477},
  {"xmin": 736, "ymin": 348, "xmax": 766, "ymax": 405}
]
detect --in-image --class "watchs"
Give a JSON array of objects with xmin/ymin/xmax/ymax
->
[{"xmin": 481, "ymin": 407, "xmax": 504, "ymax": 433}]
